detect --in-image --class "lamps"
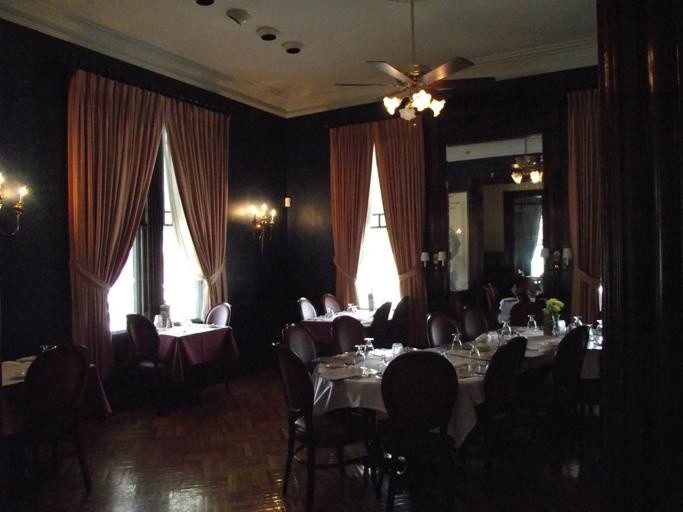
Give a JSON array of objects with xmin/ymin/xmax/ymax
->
[
  {"xmin": 382, "ymin": 1, "xmax": 446, "ymax": 123},
  {"xmin": 420, "ymin": 251, "xmax": 448, "ymax": 272},
  {"xmin": 541, "ymin": 248, "xmax": 573, "ymax": 272},
  {"xmin": 250, "ymin": 203, "xmax": 278, "ymax": 257},
  {"xmin": 510, "ymin": 135, "xmax": 544, "ymax": 184},
  {"xmin": 197, "ymin": 0, "xmax": 304, "ymax": 54},
  {"xmin": 0, "ymin": 173, "xmax": 28, "ymax": 238}
]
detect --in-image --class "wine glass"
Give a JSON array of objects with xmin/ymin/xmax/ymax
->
[
  {"xmin": 355, "ymin": 315, "xmax": 603, "ymax": 368},
  {"xmin": 346, "ymin": 303, "xmax": 352, "ymax": 312}
]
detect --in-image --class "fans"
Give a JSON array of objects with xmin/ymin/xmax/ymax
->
[{"xmin": 333, "ymin": 0, "xmax": 495, "ymax": 128}]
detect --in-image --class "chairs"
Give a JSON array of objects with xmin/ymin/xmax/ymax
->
[
  {"xmin": 0, "ymin": 343, "xmax": 93, "ymax": 511},
  {"xmin": 205, "ymin": 302, "xmax": 232, "ymax": 394},
  {"xmin": 122, "ymin": 315, "xmax": 172, "ymax": 415}
]
